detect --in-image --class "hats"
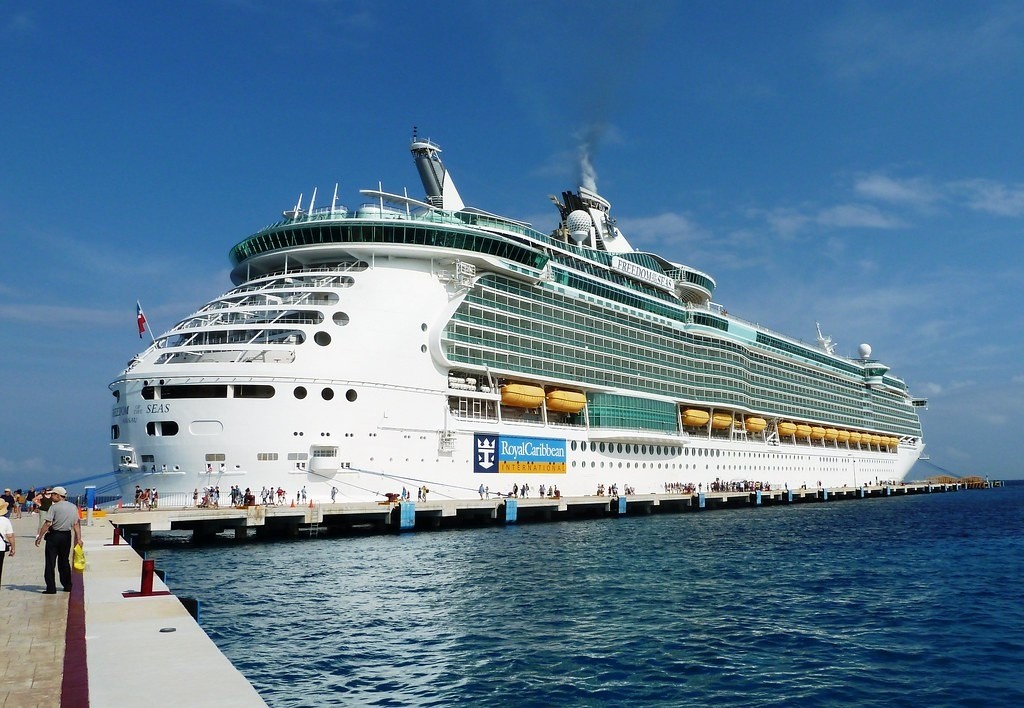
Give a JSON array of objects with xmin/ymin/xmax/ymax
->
[
  {"xmin": 46, "ymin": 486, "xmax": 68, "ymax": 497},
  {"xmin": 5, "ymin": 488, "xmax": 11, "ymax": 492},
  {"xmin": 0, "ymin": 498, "xmax": 9, "ymax": 515}
]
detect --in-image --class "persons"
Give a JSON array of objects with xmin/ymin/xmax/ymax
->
[
  {"xmin": 0, "ymin": 476, "xmax": 992, "ymax": 521},
  {"xmin": 35, "ymin": 485, "xmax": 84, "ymax": 595},
  {"xmin": 0, "ymin": 498, "xmax": 15, "ymax": 589}
]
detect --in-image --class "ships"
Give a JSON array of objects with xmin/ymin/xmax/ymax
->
[{"xmin": 108, "ymin": 125, "xmax": 930, "ymax": 533}]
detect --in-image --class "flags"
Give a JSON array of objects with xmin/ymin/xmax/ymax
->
[{"xmin": 136, "ymin": 303, "xmax": 146, "ymax": 339}]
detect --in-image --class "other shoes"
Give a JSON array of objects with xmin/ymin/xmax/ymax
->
[
  {"xmin": 63, "ymin": 588, "xmax": 71, "ymax": 591},
  {"xmin": 42, "ymin": 590, "xmax": 56, "ymax": 594}
]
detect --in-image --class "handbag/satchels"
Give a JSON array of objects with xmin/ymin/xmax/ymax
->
[
  {"xmin": 73, "ymin": 543, "xmax": 85, "ymax": 574},
  {"xmin": 0, "ymin": 533, "xmax": 11, "ymax": 551}
]
